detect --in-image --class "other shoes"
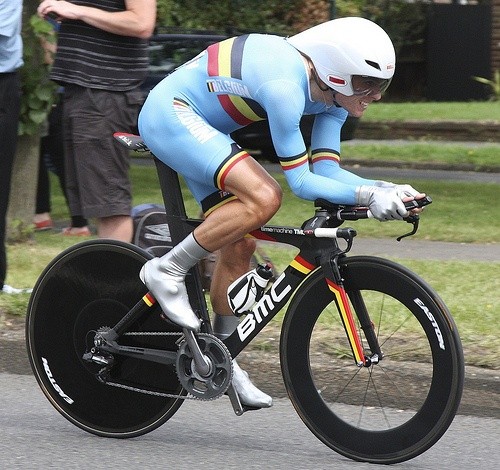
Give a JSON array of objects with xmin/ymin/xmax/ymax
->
[
  {"xmin": 32, "ymin": 219, "xmax": 53, "ymax": 230},
  {"xmin": 61, "ymin": 227, "xmax": 91, "ymax": 236}
]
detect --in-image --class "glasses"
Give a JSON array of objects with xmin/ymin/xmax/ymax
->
[{"xmin": 354, "ymin": 77, "xmax": 389, "ymax": 94}]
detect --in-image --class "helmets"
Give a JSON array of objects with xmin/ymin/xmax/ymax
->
[{"xmin": 287, "ymin": 17, "xmax": 396, "ymax": 96}]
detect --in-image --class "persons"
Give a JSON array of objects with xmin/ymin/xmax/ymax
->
[
  {"xmin": 29, "ymin": 0, "xmax": 91, "ymax": 238},
  {"xmin": 0, "ymin": 0, "xmax": 28, "ymax": 294},
  {"xmin": 37, "ymin": 0, "xmax": 158, "ymax": 244},
  {"xmin": 137, "ymin": 17, "xmax": 427, "ymax": 408}
]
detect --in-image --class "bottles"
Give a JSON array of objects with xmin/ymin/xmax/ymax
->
[{"xmin": 227, "ymin": 263, "xmax": 273, "ymax": 317}]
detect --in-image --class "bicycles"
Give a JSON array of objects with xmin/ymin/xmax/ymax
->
[{"xmin": 24, "ymin": 132, "xmax": 466, "ymax": 465}]
